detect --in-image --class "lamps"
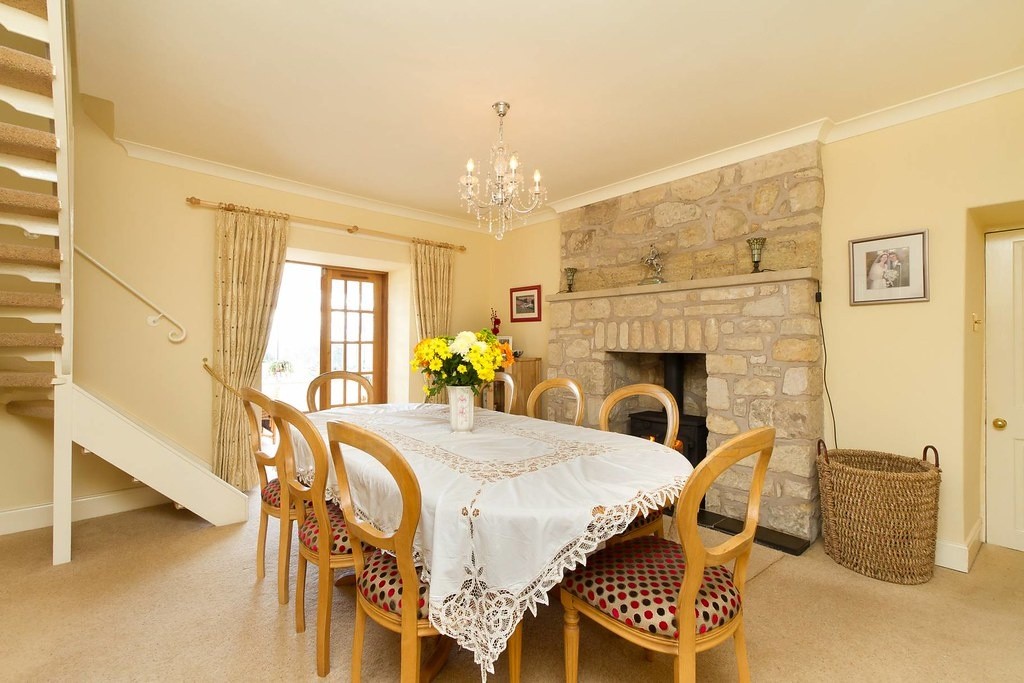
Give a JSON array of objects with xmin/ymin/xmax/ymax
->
[{"xmin": 458, "ymin": 101, "xmax": 541, "ymax": 241}]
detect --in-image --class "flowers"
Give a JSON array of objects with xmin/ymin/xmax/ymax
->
[
  {"xmin": 882, "ymin": 270, "xmax": 900, "ymax": 287},
  {"xmin": 411, "ymin": 328, "xmax": 514, "ymax": 404}
]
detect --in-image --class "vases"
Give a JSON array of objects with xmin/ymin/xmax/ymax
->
[{"xmin": 446, "ymin": 385, "xmax": 478, "ymax": 433}]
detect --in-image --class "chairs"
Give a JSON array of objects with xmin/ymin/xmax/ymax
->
[
  {"xmin": 599, "ymin": 382, "xmax": 680, "ymax": 545},
  {"xmin": 525, "ymin": 377, "xmax": 585, "ymax": 427},
  {"xmin": 327, "ymin": 419, "xmax": 452, "ymax": 683},
  {"xmin": 474, "ymin": 372, "xmax": 517, "ymax": 414},
  {"xmin": 306, "ymin": 371, "xmax": 375, "ymax": 412},
  {"xmin": 270, "ymin": 399, "xmax": 376, "ymax": 676},
  {"xmin": 240, "ymin": 387, "xmax": 307, "ymax": 603},
  {"xmin": 558, "ymin": 426, "xmax": 776, "ymax": 683}
]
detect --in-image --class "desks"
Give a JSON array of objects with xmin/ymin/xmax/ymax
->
[
  {"xmin": 475, "ymin": 356, "xmax": 542, "ymax": 418},
  {"xmin": 291, "ymin": 403, "xmax": 693, "ymax": 683}
]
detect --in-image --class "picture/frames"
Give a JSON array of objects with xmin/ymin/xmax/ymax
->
[
  {"xmin": 848, "ymin": 229, "xmax": 930, "ymax": 306},
  {"xmin": 497, "ymin": 335, "xmax": 513, "ymax": 351},
  {"xmin": 509, "ymin": 285, "xmax": 542, "ymax": 323}
]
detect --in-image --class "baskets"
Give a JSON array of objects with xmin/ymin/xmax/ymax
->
[{"xmin": 816, "ymin": 439, "xmax": 941, "ymax": 585}]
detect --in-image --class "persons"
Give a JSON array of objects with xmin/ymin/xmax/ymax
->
[{"xmin": 868, "ymin": 253, "xmax": 908, "ymax": 289}]
